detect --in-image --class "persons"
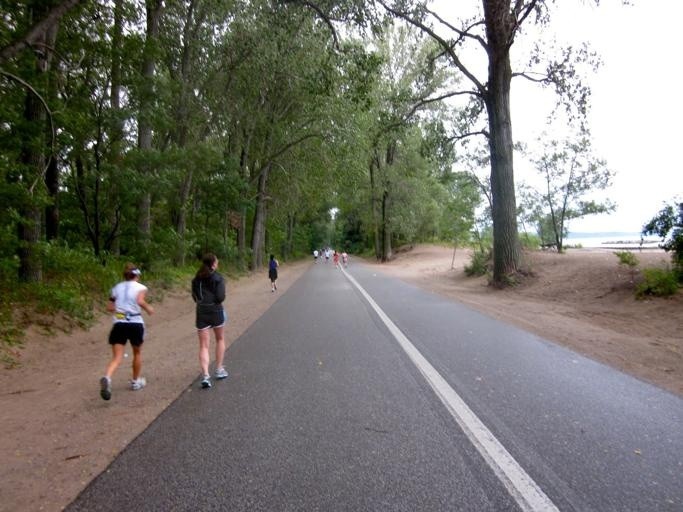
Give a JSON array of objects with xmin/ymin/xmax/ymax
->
[
  {"xmin": 313, "ymin": 247, "xmax": 348, "ymax": 269},
  {"xmin": 268, "ymin": 254, "xmax": 280, "ymax": 292},
  {"xmin": 97, "ymin": 262, "xmax": 153, "ymax": 401},
  {"xmin": 190, "ymin": 253, "xmax": 228, "ymax": 387}
]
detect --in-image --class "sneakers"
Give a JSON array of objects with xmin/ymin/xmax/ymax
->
[
  {"xmin": 131, "ymin": 376, "xmax": 145, "ymax": 390},
  {"xmin": 201, "ymin": 374, "xmax": 212, "ymax": 387},
  {"xmin": 99, "ymin": 375, "xmax": 111, "ymax": 400},
  {"xmin": 214, "ymin": 369, "xmax": 228, "ymax": 379}
]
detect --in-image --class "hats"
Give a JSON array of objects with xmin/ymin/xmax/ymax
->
[{"xmin": 131, "ymin": 268, "xmax": 141, "ymax": 275}]
